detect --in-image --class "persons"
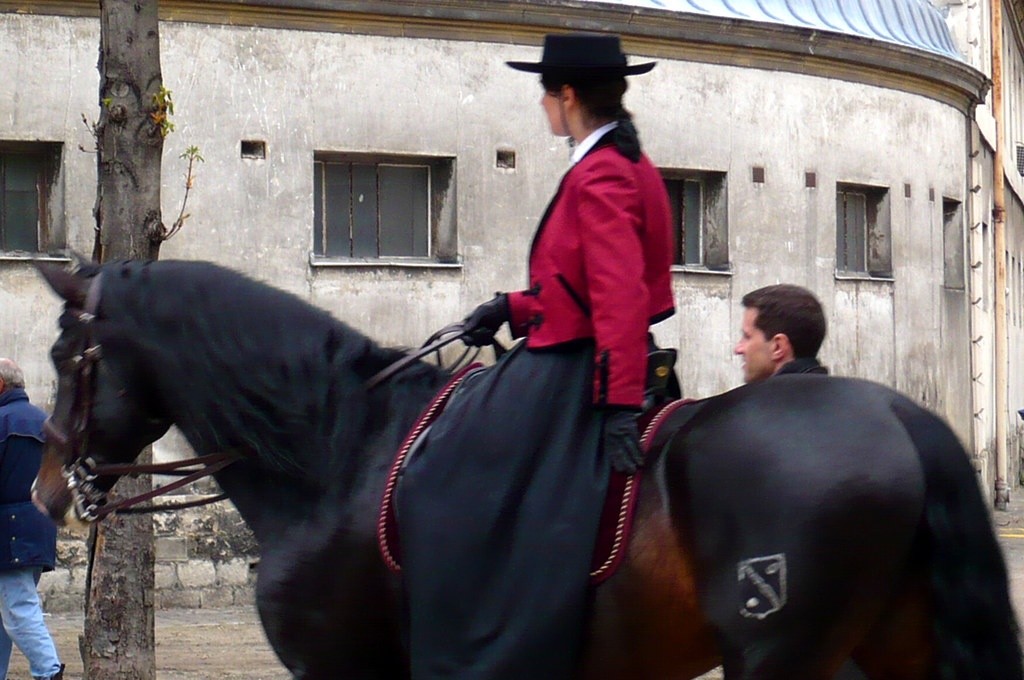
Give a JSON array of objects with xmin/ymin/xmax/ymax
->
[
  {"xmin": 0, "ymin": 357, "xmax": 66, "ymax": 680},
  {"xmin": 394, "ymin": 27, "xmax": 676, "ymax": 680},
  {"xmin": 733, "ymin": 282, "xmax": 829, "ymax": 382}
]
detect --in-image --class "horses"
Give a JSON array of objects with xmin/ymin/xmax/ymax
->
[{"xmin": 30, "ymin": 246, "xmax": 1023, "ymax": 679}]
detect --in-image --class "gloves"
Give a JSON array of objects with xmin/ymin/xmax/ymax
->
[
  {"xmin": 604, "ymin": 408, "xmax": 648, "ymax": 477},
  {"xmin": 460, "ymin": 297, "xmax": 514, "ymax": 349}
]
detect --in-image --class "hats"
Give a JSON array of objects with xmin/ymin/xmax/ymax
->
[{"xmin": 504, "ymin": 33, "xmax": 656, "ymax": 76}]
